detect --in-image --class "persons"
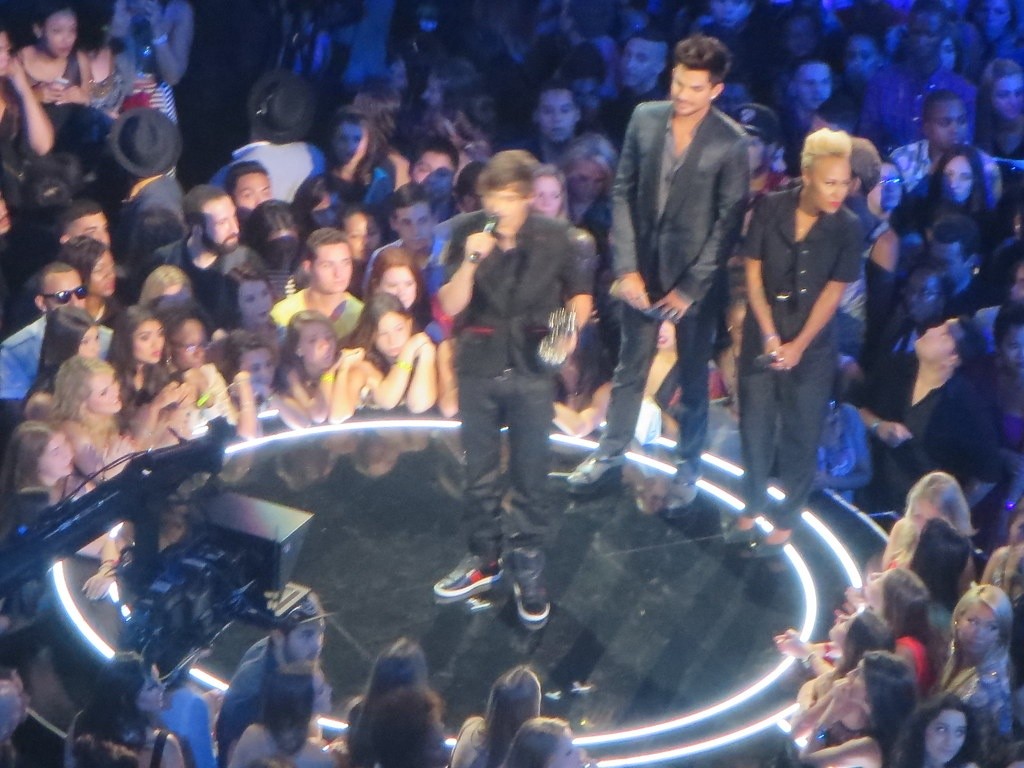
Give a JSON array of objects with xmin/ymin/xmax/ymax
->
[
  {"xmin": 0, "ymin": 521, "xmax": 603, "ymax": 768},
  {"xmin": 774, "ymin": 470, "xmax": 1024, "ymax": 768},
  {"xmin": 71, "ymin": 428, "xmax": 677, "ymax": 600},
  {"xmin": 670, "ymin": 0, "xmax": 1024, "ymax": 520},
  {"xmin": 567, "ymin": 36, "xmax": 750, "ymax": 519},
  {"xmin": 0, "ymin": 0, "xmax": 670, "ymax": 522},
  {"xmin": 434, "ymin": 149, "xmax": 598, "ymax": 625},
  {"xmin": 723, "ymin": 127, "xmax": 861, "ymax": 562}
]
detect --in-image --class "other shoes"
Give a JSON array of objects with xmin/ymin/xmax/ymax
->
[
  {"xmin": 567, "ymin": 454, "xmax": 626, "ymax": 487},
  {"xmin": 664, "ymin": 484, "xmax": 699, "ymax": 517}
]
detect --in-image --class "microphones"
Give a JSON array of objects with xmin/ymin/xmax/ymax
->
[
  {"xmin": 754, "ymin": 354, "xmax": 775, "ymax": 366},
  {"xmin": 470, "ymin": 215, "xmax": 499, "ymax": 260}
]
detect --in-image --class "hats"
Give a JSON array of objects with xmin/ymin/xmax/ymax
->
[
  {"xmin": 246, "ymin": 71, "xmax": 317, "ymax": 146},
  {"xmin": 732, "ymin": 103, "xmax": 778, "ymax": 137},
  {"xmin": 264, "ymin": 586, "xmax": 332, "ymax": 629},
  {"xmin": 110, "ymin": 107, "xmax": 181, "ymax": 178},
  {"xmin": 23, "ymin": 153, "xmax": 92, "ymax": 211},
  {"xmin": 850, "ymin": 137, "xmax": 882, "ymax": 195}
]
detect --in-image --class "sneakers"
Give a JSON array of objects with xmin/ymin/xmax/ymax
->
[
  {"xmin": 434, "ymin": 559, "xmax": 503, "ymax": 597},
  {"xmin": 512, "ymin": 583, "xmax": 551, "ymax": 622}
]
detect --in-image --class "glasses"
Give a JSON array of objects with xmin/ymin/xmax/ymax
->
[
  {"xmin": 168, "ymin": 341, "xmax": 215, "ymax": 354},
  {"xmin": 44, "ymin": 287, "xmax": 87, "ymax": 305},
  {"xmin": 904, "ymin": 288, "xmax": 945, "ymax": 302},
  {"xmin": 880, "ymin": 177, "xmax": 904, "ymax": 187}
]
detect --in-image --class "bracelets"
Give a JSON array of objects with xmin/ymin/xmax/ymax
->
[
  {"xmin": 321, "ymin": 372, "xmax": 335, "ymax": 386},
  {"xmin": 815, "ymin": 727, "xmax": 828, "ymax": 742},
  {"xmin": 801, "ymin": 651, "xmax": 820, "ymax": 673},
  {"xmin": 872, "ymin": 418, "xmax": 883, "ymax": 437},
  {"xmin": 763, "ymin": 333, "xmax": 784, "ymax": 344},
  {"xmin": 394, "ymin": 360, "xmax": 414, "ymax": 374}
]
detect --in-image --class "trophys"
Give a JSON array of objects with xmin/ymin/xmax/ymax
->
[{"xmin": 539, "ymin": 307, "xmax": 579, "ymax": 366}]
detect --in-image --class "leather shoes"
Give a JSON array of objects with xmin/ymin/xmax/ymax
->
[
  {"xmin": 750, "ymin": 527, "xmax": 798, "ymax": 558},
  {"xmin": 725, "ymin": 512, "xmax": 758, "ymax": 541}
]
